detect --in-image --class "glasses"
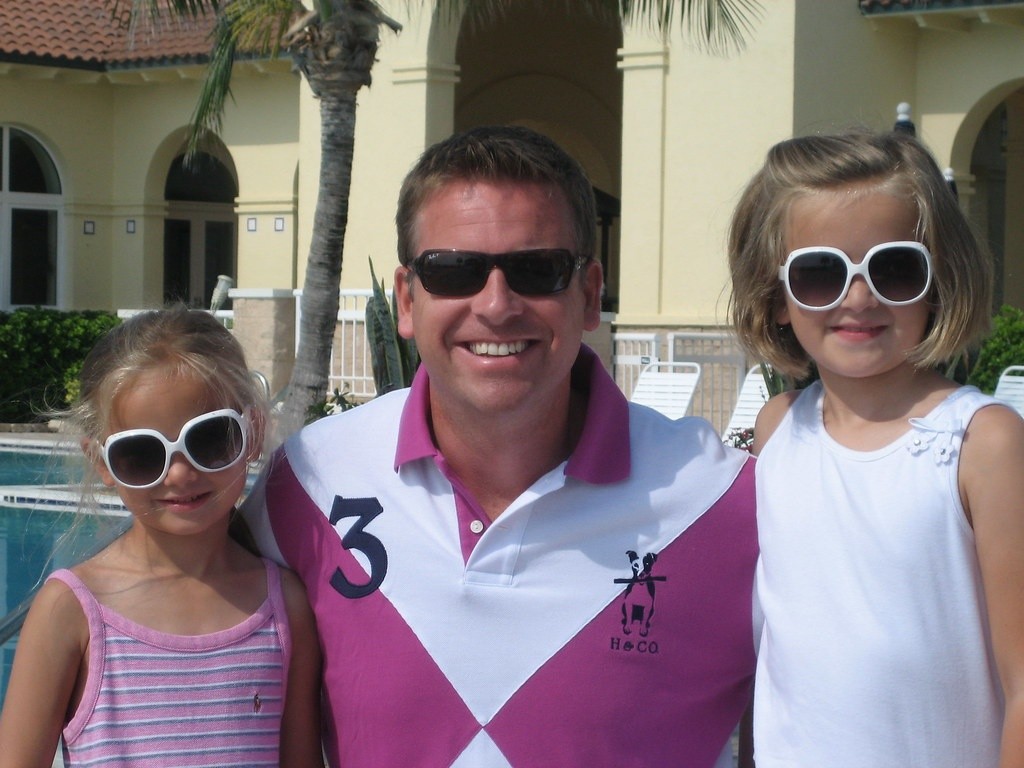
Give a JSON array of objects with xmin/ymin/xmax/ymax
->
[
  {"xmin": 777, "ymin": 241, "xmax": 934, "ymax": 312},
  {"xmin": 92, "ymin": 407, "xmax": 248, "ymax": 489},
  {"xmin": 407, "ymin": 248, "xmax": 589, "ymax": 297}
]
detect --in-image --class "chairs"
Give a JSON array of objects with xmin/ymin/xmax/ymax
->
[
  {"xmin": 631, "ymin": 360, "xmax": 700, "ymax": 420},
  {"xmin": 994, "ymin": 365, "xmax": 1024, "ymax": 419},
  {"xmin": 723, "ymin": 362, "xmax": 772, "ymax": 452}
]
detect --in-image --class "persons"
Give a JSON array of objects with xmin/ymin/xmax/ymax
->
[
  {"xmin": 226, "ymin": 129, "xmax": 760, "ymax": 768},
  {"xmin": 729, "ymin": 131, "xmax": 1024, "ymax": 768},
  {"xmin": 1, "ymin": 304, "xmax": 322, "ymax": 768}
]
